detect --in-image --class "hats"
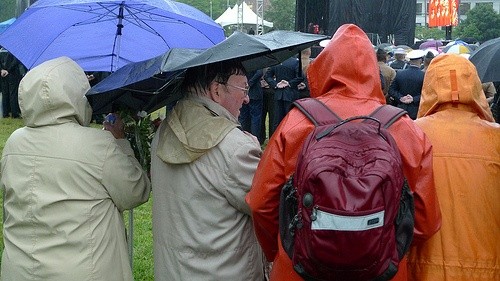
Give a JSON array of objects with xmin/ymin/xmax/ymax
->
[
  {"xmin": 407, "ymin": 50, "xmax": 425, "ymax": 60},
  {"xmin": 377, "ymin": 47, "xmax": 386, "ymax": 54},
  {"xmin": 424, "ymin": 49, "xmax": 439, "ymax": 58},
  {"xmin": 320, "ymin": 39, "xmax": 331, "ymax": 47},
  {"xmin": 395, "ymin": 48, "xmax": 406, "ymax": 54}
]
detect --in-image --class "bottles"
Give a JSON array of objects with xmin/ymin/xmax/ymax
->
[{"xmin": 102, "ymin": 113, "xmax": 115, "ymax": 132}]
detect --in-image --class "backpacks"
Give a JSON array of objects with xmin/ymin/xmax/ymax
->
[{"xmin": 279, "ymin": 97, "xmax": 414, "ymax": 281}]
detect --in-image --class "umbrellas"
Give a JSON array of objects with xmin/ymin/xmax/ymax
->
[
  {"xmin": 86, "ymin": 31, "xmax": 331, "ymax": 116},
  {"xmin": 0, "ymin": 0, "xmax": 226, "ymax": 124},
  {"xmin": 418, "ymin": 39, "xmax": 500, "ymax": 83}
]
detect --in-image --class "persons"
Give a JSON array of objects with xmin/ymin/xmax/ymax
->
[
  {"xmin": 245, "ymin": 24, "xmax": 442, "ymax": 281},
  {"xmin": 375, "ymin": 48, "xmax": 439, "ymax": 120},
  {"xmin": 0, "ymin": 56, "xmax": 151, "ymax": 281},
  {"xmin": 238, "ymin": 57, "xmax": 309, "ymax": 144},
  {"xmin": 151, "ymin": 57, "xmax": 271, "ymax": 281},
  {"xmin": 0, "ymin": 45, "xmax": 28, "ymax": 119},
  {"xmin": 406, "ymin": 55, "xmax": 500, "ymax": 281},
  {"xmin": 85, "ymin": 71, "xmax": 105, "ymax": 124}
]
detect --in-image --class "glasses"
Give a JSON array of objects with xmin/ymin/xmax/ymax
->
[{"xmin": 217, "ymin": 81, "xmax": 250, "ymax": 96}]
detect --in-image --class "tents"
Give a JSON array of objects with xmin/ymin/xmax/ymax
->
[{"xmin": 215, "ymin": 2, "xmax": 274, "ymax": 29}]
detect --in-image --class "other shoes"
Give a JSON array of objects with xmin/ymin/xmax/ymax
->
[
  {"xmin": 3, "ymin": 112, "xmax": 10, "ymax": 118},
  {"xmin": 12, "ymin": 114, "xmax": 23, "ymax": 119}
]
